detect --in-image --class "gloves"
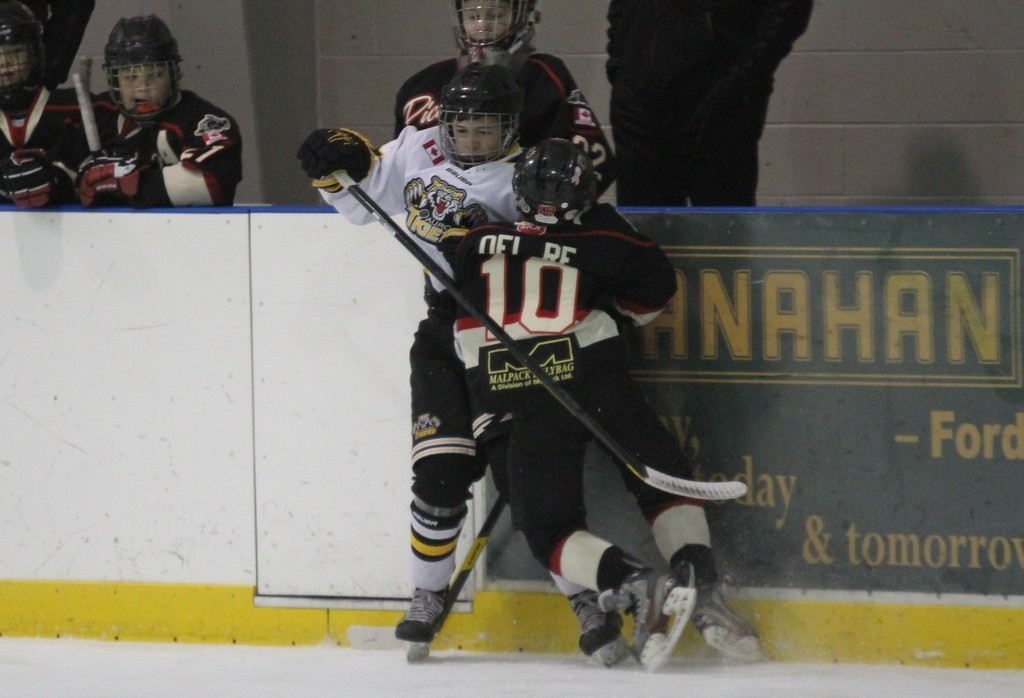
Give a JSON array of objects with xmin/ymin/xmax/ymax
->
[
  {"xmin": 0, "ymin": 147, "xmax": 58, "ymax": 207},
  {"xmin": 488, "ymin": 440, "xmax": 511, "ymax": 506},
  {"xmin": 595, "ymin": 293, "xmax": 631, "ymax": 325},
  {"xmin": 297, "ymin": 127, "xmax": 372, "ymax": 193},
  {"xmin": 76, "ymin": 147, "xmax": 140, "ymax": 208}
]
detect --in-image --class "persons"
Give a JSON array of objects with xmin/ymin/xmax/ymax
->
[
  {"xmin": 605, "ymin": 0, "xmax": 816, "ymax": 207},
  {"xmin": 0, "ymin": 0, "xmax": 241, "ymax": 207},
  {"xmin": 452, "ymin": 138, "xmax": 761, "ymax": 672},
  {"xmin": 393, "ymin": 0, "xmax": 618, "ymax": 203},
  {"xmin": 297, "ymin": 62, "xmax": 624, "ymax": 657}
]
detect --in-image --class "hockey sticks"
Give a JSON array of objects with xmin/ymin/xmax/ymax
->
[
  {"xmin": 71, "ymin": 53, "xmax": 105, "ymax": 161},
  {"xmin": 348, "ymin": 493, "xmax": 506, "ymax": 648},
  {"xmin": 335, "ymin": 172, "xmax": 749, "ymax": 500}
]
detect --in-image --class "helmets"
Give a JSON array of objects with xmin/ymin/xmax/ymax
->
[
  {"xmin": 103, "ymin": 15, "xmax": 183, "ymax": 118},
  {"xmin": 512, "ymin": 138, "xmax": 592, "ymax": 227},
  {"xmin": 0, "ymin": 0, "xmax": 44, "ymax": 102},
  {"xmin": 452, "ymin": 0, "xmax": 535, "ymax": 48},
  {"xmin": 438, "ymin": 63, "xmax": 524, "ymax": 169}
]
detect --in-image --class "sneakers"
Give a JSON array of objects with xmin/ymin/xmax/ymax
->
[
  {"xmin": 678, "ymin": 560, "xmax": 762, "ymax": 660},
  {"xmin": 396, "ymin": 588, "xmax": 447, "ymax": 662},
  {"xmin": 598, "ymin": 557, "xmax": 698, "ymax": 672},
  {"xmin": 568, "ymin": 588, "xmax": 632, "ymax": 668}
]
